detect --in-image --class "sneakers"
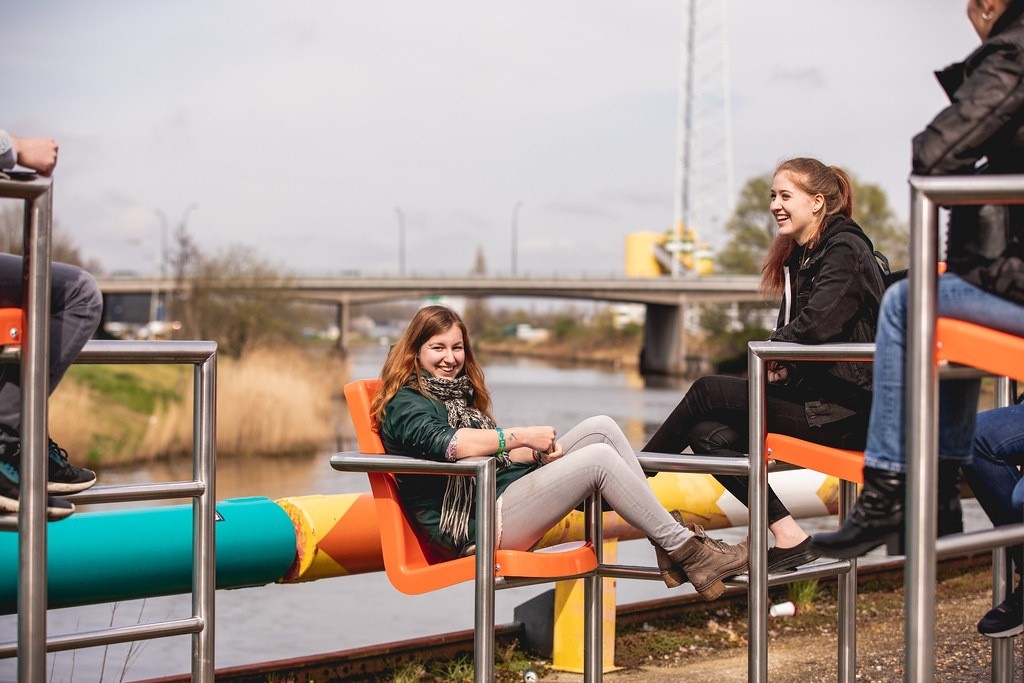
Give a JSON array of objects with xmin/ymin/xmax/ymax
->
[
  {"xmin": 4, "ymin": 436, "xmax": 96, "ymax": 494},
  {"xmin": 0, "ymin": 459, "xmax": 75, "ymax": 521},
  {"xmin": 977, "ymin": 570, "xmax": 1024, "ymax": 637}
]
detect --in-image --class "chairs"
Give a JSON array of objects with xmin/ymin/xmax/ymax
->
[
  {"xmin": 764, "ymin": 317, "xmax": 1023, "ymax": 500},
  {"xmin": 344, "ymin": 378, "xmax": 599, "ymax": 596}
]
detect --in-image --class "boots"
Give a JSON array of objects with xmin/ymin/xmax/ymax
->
[
  {"xmin": 647, "ymin": 509, "xmax": 689, "ymax": 589},
  {"xmin": 668, "ymin": 522, "xmax": 749, "ymax": 601}
]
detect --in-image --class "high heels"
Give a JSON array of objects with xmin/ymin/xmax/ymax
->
[
  {"xmin": 938, "ymin": 457, "xmax": 965, "ymax": 536},
  {"xmin": 807, "ymin": 466, "xmax": 906, "ymax": 560}
]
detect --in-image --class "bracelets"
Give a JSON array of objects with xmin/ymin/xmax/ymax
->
[
  {"xmin": 532, "ymin": 449, "xmax": 541, "ymax": 463},
  {"xmin": 495, "ymin": 427, "xmax": 506, "ymax": 457}
]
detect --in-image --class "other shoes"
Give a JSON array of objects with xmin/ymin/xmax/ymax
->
[
  {"xmin": 575, "ymin": 495, "xmax": 613, "ymax": 512},
  {"xmin": 768, "ymin": 535, "xmax": 822, "ymax": 573}
]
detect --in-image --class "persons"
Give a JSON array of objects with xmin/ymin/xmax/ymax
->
[
  {"xmin": 1, "ymin": 129, "xmax": 105, "ymax": 520},
  {"xmin": 370, "ymin": 306, "xmax": 749, "ymax": 601},
  {"xmin": 575, "ymin": 158, "xmax": 886, "ymax": 574},
  {"xmin": 962, "ymin": 404, "xmax": 1024, "ymax": 638},
  {"xmin": 808, "ymin": 0, "xmax": 1024, "ymax": 561}
]
